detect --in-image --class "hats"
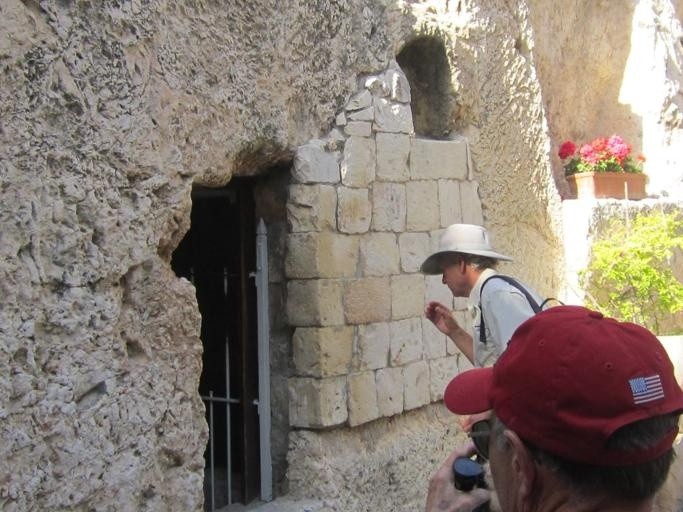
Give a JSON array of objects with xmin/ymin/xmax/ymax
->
[
  {"xmin": 420, "ymin": 224, "xmax": 514, "ymax": 274},
  {"xmin": 445, "ymin": 306, "xmax": 683, "ymax": 466}
]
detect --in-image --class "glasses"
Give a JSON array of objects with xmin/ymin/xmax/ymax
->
[{"xmin": 467, "ymin": 420, "xmax": 541, "ymax": 466}]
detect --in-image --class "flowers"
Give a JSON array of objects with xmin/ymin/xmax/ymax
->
[{"xmin": 558, "ymin": 134, "xmax": 646, "ymax": 177}]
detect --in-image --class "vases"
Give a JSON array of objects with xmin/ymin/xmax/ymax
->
[{"xmin": 565, "ymin": 173, "xmax": 649, "ymax": 200}]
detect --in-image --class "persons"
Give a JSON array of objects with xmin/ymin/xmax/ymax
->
[
  {"xmin": 419, "ymin": 223, "xmax": 552, "ymax": 368},
  {"xmin": 425, "ymin": 300, "xmax": 683, "ymax": 512}
]
